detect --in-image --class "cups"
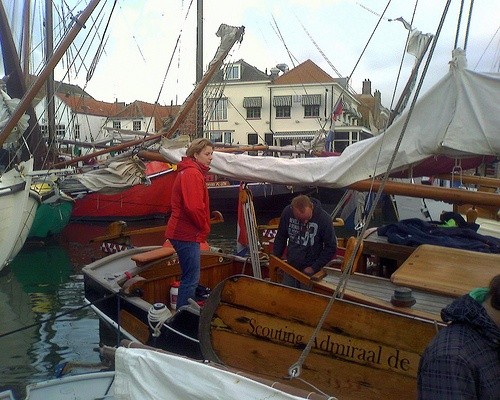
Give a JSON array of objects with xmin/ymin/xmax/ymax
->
[{"xmin": 393, "ymin": 287, "xmax": 412, "ymax": 303}]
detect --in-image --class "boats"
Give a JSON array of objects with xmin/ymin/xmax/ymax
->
[{"xmin": 0, "ymin": 338, "xmax": 338, "ymax": 400}]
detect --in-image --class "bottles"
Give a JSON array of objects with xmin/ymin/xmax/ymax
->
[{"xmin": 170, "ymin": 282, "xmax": 178, "ymax": 310}]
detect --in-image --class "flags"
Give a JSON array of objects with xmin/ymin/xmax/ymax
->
[
  {"xmin": 236, "ymin": 188, "xmax": 250, "ymax": 255},
  {"xmin": 325, "ymin": 99, "xmax": 343, "ymax": 149}
]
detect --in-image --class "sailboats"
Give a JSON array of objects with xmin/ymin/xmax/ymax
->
[{"xmin": 0, "ymin": 0, "xmax": 500, "ymax": 399}]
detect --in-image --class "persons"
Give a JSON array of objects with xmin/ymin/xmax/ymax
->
[
  {"xmin": 166, "ymin": 137, "xmax": 213, "ymax": 312},
  {"xmin": 417, "ymin": 274, "xmax": 500, "ymax": 400},
  {"xmin": 274, "ymin": 195, "xmax": 337, "ymax": 292}
]
